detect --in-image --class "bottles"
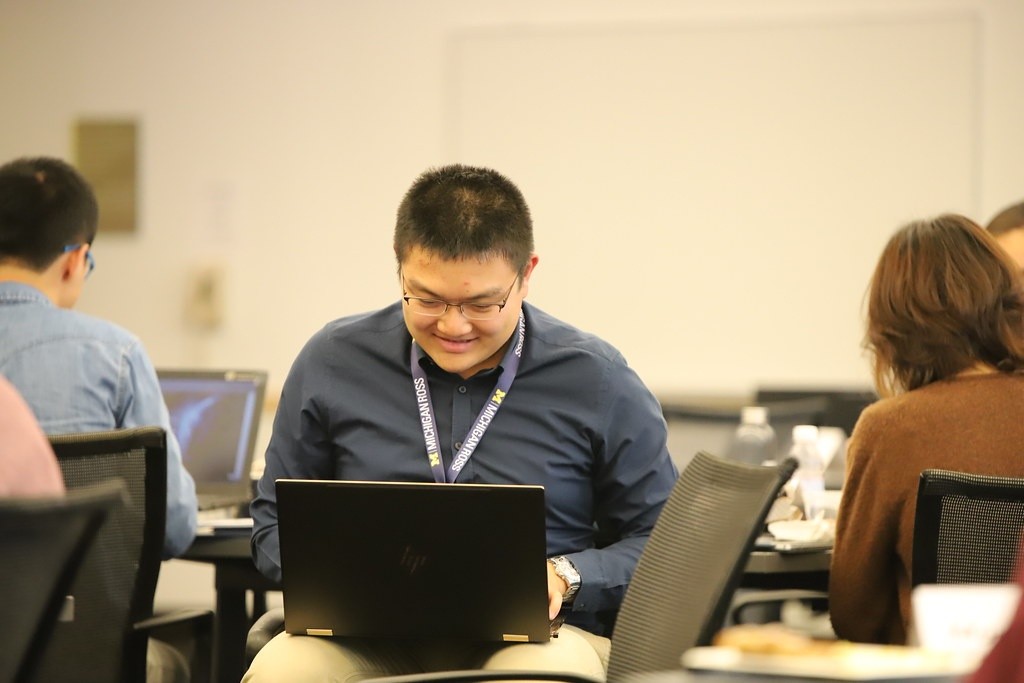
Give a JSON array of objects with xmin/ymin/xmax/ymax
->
[
  {"xmin": 789, "ymin": 425, "xmax": 827, "ymax": 520},
  {"xmin": 731, "ymin": 406, "xmax": 779, "ymax": 469}
]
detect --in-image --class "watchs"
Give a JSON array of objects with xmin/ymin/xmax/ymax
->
[{"xmin": 548, "ymin": 556, "xmax": 581, "ymax": 603}]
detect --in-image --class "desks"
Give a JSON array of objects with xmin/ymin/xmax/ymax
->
[{"xmin": 189, "ymin": 535, "xmax": 283, "ymax": 683}]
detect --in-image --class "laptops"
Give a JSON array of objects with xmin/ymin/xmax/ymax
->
[
  {"xmin": 275, "ymin": 478, "xmax": 567, "ymax": 642},
  {"xmin": 154, "ymin": 368, "xmax": 267, "ymax": 513}
]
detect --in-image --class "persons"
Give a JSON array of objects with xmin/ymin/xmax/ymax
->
[
  {"xmin": 0, "ymin": 156, "xmax": 198, "ymax": 563},
  {"xmin": 826, "ymin": 215, "xmax": 1024, "ymax": 648},
  {"xmin": 238, "ymin": 164, "xmax": 680, "ymax": 683}
]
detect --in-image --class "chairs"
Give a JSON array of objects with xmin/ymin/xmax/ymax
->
[
  {"xmin": 49, "ymin": 425, "xmax": 211, "ymax": 683},
  {"xmin": 912, "ymin": 469, "xmax": 1024, "ymax": 593},
  {"xmin": 604, "ymin": 451, "xmax": 802, "ymax": 683}
]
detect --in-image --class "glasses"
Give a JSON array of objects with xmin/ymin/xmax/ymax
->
[
  {"xmin": 401, "ymin": 265, "xmax": 521, "ymax": 320},
  {"xmin": 65, "ymin": 244, "xmax": 95, "ymax": 279}
]
